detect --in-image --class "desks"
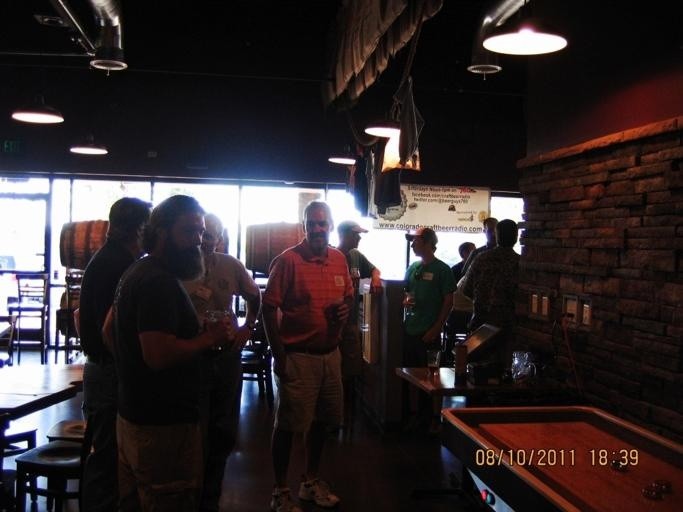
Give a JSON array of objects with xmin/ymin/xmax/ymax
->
[
  {"xmin": 434, "ymin": 404, "xmax": 682, "ymax": 512},
  {"xmin": 393, "ymin": 361, "xmax": 567, "ymax": 465}
]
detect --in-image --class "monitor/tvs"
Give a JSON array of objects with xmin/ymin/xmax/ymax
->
[{"xmin": 451, "ymin": 322, "xmax": 504, "ymax": 361}]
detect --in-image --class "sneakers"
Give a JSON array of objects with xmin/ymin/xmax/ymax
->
[
  {"xmin": 298, "ymin": 478, "xmax": 343, "ymax": 508},
  {"xmin": 270, "ymin": 484, "xmax": 304, "ymax": 512}
]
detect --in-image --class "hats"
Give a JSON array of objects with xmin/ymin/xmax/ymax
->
[
  {"xmin": 406, "ymin": 228, "xmax": 436, "ymax": 244},
  {"xmin": 337, "ymin": 221, "xmax": 368, "ymax": 233}
]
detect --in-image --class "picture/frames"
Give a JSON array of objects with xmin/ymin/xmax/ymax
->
[{"xmin": 525, "ymin": 288, "xmax": 593, "ymax": 331}]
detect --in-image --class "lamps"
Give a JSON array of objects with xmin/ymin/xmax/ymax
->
[
  {"xmin": 326, "ymin": 142, "xmax": 355, "ymax": 166},
  {"xmin": 65, "ymin": 99, "xmax": 108, "ymax": 158},
  {"xmin": 479, "ymin": 0, "xmax": 569, "ymax": 60},
  {"xmin": 10, "ymin": 57, "xmax": 65, "ymax": 127},
  {"xmin": 365, "ymin": 116, "xmax": 402, "ymax": 143}
]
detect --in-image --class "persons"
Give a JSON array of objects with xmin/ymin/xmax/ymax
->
[
  {"xmin": 335, "ymin": 220, "xmax": 383, "ymax": 421},
  {"xmin": 262, "ymin": 201, "xmax": 355, "ymax": 511},
  {"xmin": 71, "ymin": 195, "xmax": 261, "ymax": 512},
  {"xmin": 401, "ymin": 217, "xmax": 522, "ymax": 435}
]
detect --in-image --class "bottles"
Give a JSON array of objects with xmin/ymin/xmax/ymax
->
[{"xmin": 453, "ymin": 344, "xmax": 468, "ymax": 387}]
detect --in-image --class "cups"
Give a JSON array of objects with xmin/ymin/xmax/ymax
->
[
  {"xmin": 511, "ymin": 349, "xmax": 536, "ymax": 385},
  {"xmin": 424, "ymin": 349, "xmax": 441, "ymax": 376},
  {"xmin": 405, "ymin": 292, "xmax": 417, "ymax": 317},
  {"xmin": 203, "ymin": 309, "xmax": 231, "ymax": 352},
  {"xmin": 324, "ymin": 296, "xmax": 345, "ymax": 339}
]
